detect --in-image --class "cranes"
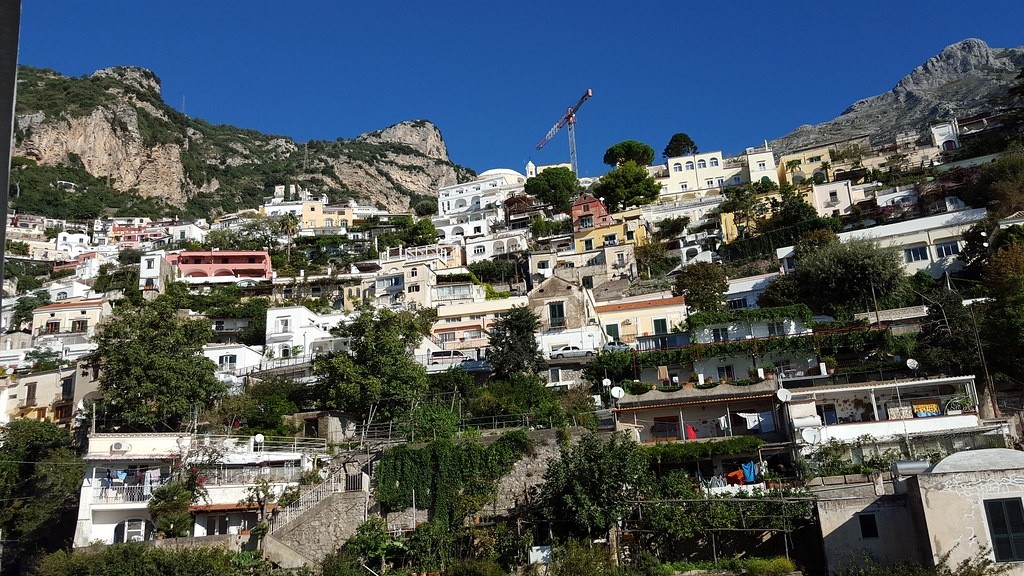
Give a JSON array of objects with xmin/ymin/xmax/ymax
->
[{"xmin": 533, "ymin": 88, "xmax": 596, "ymax": 180}]
